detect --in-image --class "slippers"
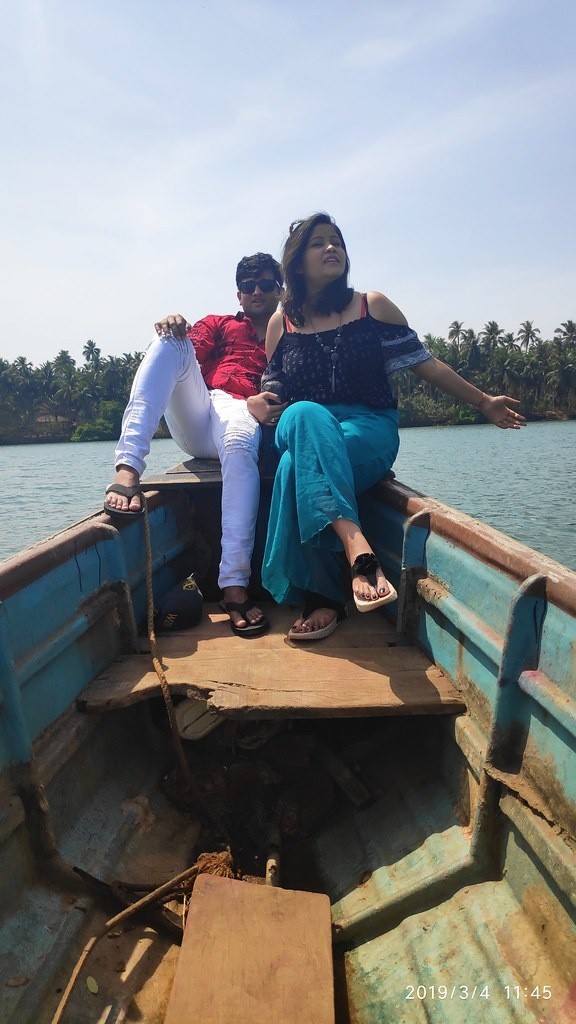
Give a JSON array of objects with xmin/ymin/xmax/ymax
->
[
  {"xmin": 103, "ymin": 483, "xmax": 145, "ymax": 517},
  {"xmin": 289, "ymin": 595, "xmax": 349, "ymax": 641},
  {"xmin": 349, "ymin": 553, "xmax": 397, "ymax": 613},
  {"xmin": 218, "ymin": 598, "xmax": 270, "ymax": 638}
]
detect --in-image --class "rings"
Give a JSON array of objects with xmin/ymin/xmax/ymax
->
[{"xmin": 269, "ymin": 418, "xmax": 276, "ymax": 423}]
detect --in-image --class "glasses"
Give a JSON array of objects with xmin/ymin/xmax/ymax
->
[{"xmin": 237, "ymin": 278, "xmax": 281, "ymax": 296}]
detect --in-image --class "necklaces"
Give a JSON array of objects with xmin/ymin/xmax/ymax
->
[{"xmin": 305, "ymin": 306, "xmax": 343, "ymax": 395}]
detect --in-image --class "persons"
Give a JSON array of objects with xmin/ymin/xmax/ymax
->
[
  {"xmin": 245, "ymin": 209, "xmax": 529, "ymax": 640},
  {"xmin": 104, "ymin": 250, "xmax": 284, "ymax": 639}
]
